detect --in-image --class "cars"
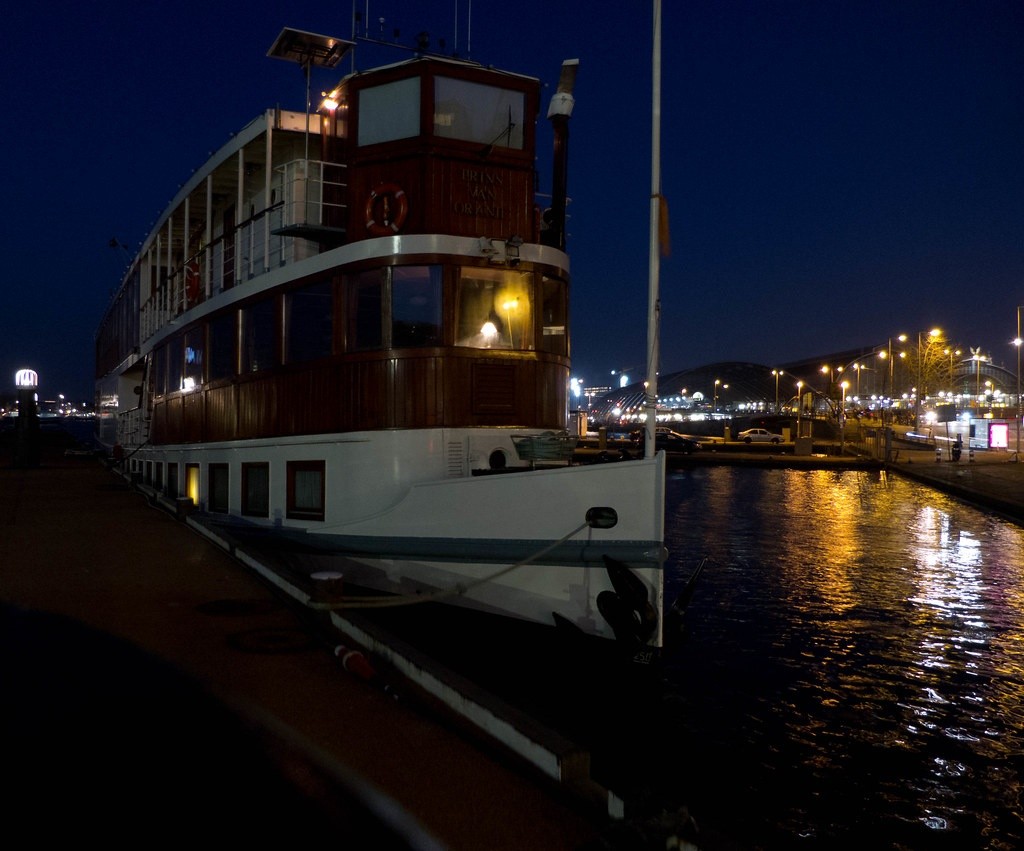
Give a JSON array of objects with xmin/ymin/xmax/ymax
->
[
  {"xmin": 637, "ymin": 427, "xmax": 702, "ymax": 453},
  {"xmin": 738, "ymin": 429, "xmax": 785, "ymax": 444},
  {"xmin": 956, "ymin": 410, "xmax": 970, "ymax": 421}
]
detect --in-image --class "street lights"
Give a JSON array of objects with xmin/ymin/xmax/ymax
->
[
  {"xmin": 715, "ymin": 380, "xmax": 720, "ymax": 413},
  {"xmin": 831, "ymin": 367, "xmax": 843, "ymax": 382},
  {"xmin": 915, "ymin": 330, "xmax": 940, "ymax": 433},
  {"xmin": 797, "ymin": 382, "xmax": 804, "ymax": 438},
  {"xmin": 772, "ymin": 370, "xmax": 783, "ymax": 407},
  {"xmin": 944, "ymin": 350, "xmax": 961, "ymax": 385},
  {"xmin": 842, "ymin": 382, "xmax": 849, "ymax": 414},
  {"xmin": 973, "ymin": 355, "xmax": 985, "ymax": 418},
  {"xmin": 890, "ymin": 353, "xmax": 905, "ymax": 401},
  {"xmin": 853, "ymin": 364, "xmax": 865, "ymax": 396},
  {"xmin": 584, "ymin": 393, "xmax": 596, "ymax": 419},
  {"xmin": 723, "ymin": 385, "xmax": 728, "ymax": 427},
  {"xmin": 888, "ymin": 335, "xmax": 907, "ymax": 374},
  {"xmin": 986, "ymin": 382, "xmax": 993, "ymax": 393}
]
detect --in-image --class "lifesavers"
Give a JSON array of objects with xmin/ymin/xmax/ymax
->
[
  {"xmin": 533, "ymin": 205, "xmax": 540, "ymax": 244},
  {"xmin": 186, "ymin": 262, "xmax": 200, "ymax": 298},
  {"xmin": 363, "ymin": 183, "xmax": 408, "ymax": 236}
]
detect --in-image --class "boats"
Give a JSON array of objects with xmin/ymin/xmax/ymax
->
[{"xmin": 93, "ymin": 0, "xmax": 666, "ymax": 683}]
